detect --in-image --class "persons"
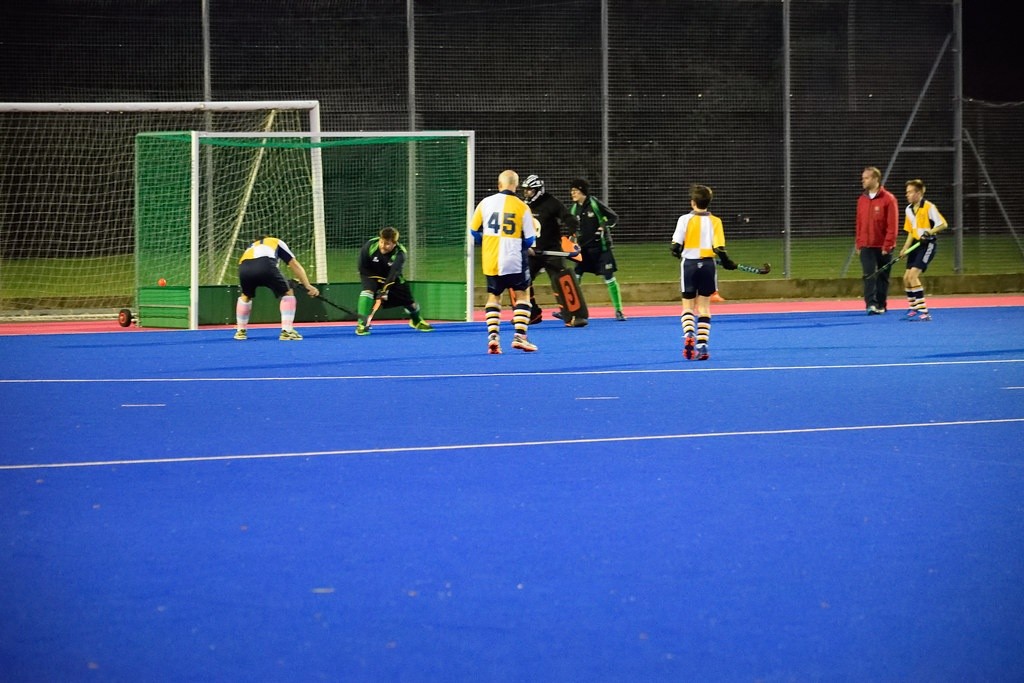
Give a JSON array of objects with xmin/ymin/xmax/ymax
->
[
  {"xmin": 469, "ymin": 170, "xmax": 538, "ymax": 354},
  {"xmin": 668, "ymin": 185, "xmax": 733, "ymax": 360},
  {"xmin": 511, "ymin": 174, "xmax": 589, "ymax": 328},
  {"xmin": 856, "ymin": 167, "xmax": 898, "ymax": 315},
  {"xmin": 233, "ymin": 236, "xmax": 319, "ymax": 340},
  {"xmin": 898, "ymin": 179, "xmax": 948, "ymax": 321},
  {"xmin": 355, "ymin": 227, "xmax": 433, "ymax": 336},
  {"xmin": 552, "ymin": 177, "xmax": 626, "ymax": 321}
]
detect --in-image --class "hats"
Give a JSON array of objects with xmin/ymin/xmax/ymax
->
[{"xmin": 569, "ymin": 178, "xmax": 590, "ymax": 196}]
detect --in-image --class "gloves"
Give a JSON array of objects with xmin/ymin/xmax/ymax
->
[
  {"xmin": 723, "ymin": 260, "xmax": 734, "ymax": 271},
  {"xmin": 920, "ymin": 232, "xmax": 931, "ymax": 245}
]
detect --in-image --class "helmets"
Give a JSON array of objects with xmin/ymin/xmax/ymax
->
[{"xmin": 521, "ymin": 175, "xmax": 545, "ymax": 205}]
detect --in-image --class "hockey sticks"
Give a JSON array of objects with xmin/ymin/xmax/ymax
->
[
  {"xmin": 531, "ymin": 242, "xmax": 582, "ymax": 257},
  {"xmin": 861, "ymin": 241, "xmax": 920, "ymax": 282},
  {"xmin": 713, "ymin": 258, "xmax": 772, "ymax": 275},
  {"xmin": 287, "ymin": 279, "xmax": 368, "ymax": 326}
]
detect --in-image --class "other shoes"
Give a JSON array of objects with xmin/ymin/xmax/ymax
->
[
  {"xmin": 355, "ymin": 324, "xmax": 370, "ymax": 335},
  {"xmin": 617, "ymin": 310, "xmax": 625, "ymax": 320},
  {"xmin": 234, "ymin": 329, "xmax": 247, "ymax": 340},
  {"xmin": 279, "ymin": 329, "xmax": 303, "ymax": 341},
  {"xmin": 511, "ymin": 314, "xmax": 542, "ymax": 324},
  {"xmin": 866, "ymin": 305, "xmax": 885, "ymax": 315},
  {"xmin": 553, "ymin": 311, "xmax": 563, "ymax": 318},
  {"xmin": 410, "ymin": 319, "xmax": 433, "ymax": 331}
]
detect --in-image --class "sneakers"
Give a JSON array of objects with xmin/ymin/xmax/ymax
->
[
  {"xmin": 909, "ymin": 312, "xmax": 931, "ymax": 321},
  {"xmin": 512, "ymin": 333, "xmax": 537, "ymax": 351},
  {"xmin": 695, "ymin": 344, "xmax": 708, "ymax": 359},
  {"xmin": 487, "ymin": 333, "xmax": 502, "ymax": 354},
  {"xmin": 898, "ymin": 310, "xmax": 918, "ymax": 320},
  {"xmin": 682, "ymin": 332, "xmax": 695, "ymax": 360}
]
